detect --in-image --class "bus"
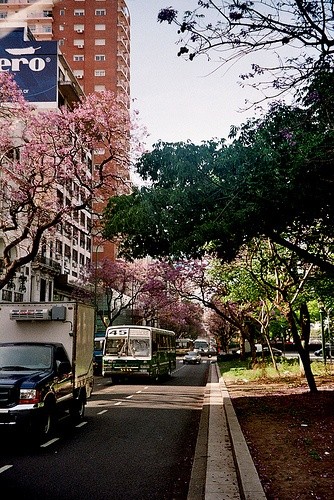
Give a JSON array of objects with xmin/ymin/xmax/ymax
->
[
  {"xmin": 175, "ymin": 339, "xmax": 193, "ymax": 354},
  {"xmin": 102, "ymin": 326, "xmax": 178, "ymax": 382},
  {"xmin": 193, "ymin": 340, "xmax": 210, "ymax": 355}
]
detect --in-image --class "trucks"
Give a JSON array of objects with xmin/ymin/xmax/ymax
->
[{"xmin": 0, "ymin": 302, "xmax": 97, "ymax": 434}]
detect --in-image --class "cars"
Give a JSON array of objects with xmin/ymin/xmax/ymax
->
[
  {"xmin": 315, "ymin": 345, "xmax": 334, "ymax": 356},
  {"xmin": 183, "ymin": 351, "xmax": 202, "ymax": 363},
  {"xmin": 261, "ymin": 348, "xmax": 283, "ymax": 357}
]
